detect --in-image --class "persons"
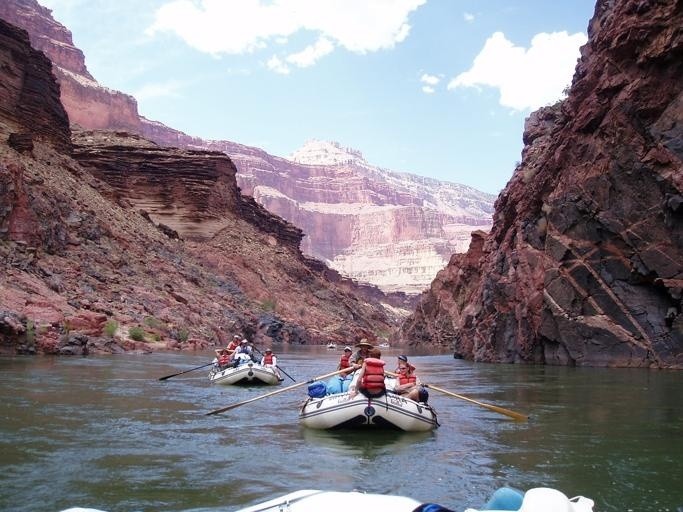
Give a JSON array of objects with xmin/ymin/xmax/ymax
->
[
  {"xmin": 348, "ymin": 338, "xmax": 373, "ymax": 371},
  {"xmin": 327, "ymin": 340, "xmax": 335, "ymax": 345},
  {"xmin": 214, "ymin": 334, "xmax": 284, "ymax": 382},
  {"xmin": 394, "ymin": 354, "xmax": 415, "ymax": 374},
  {"xmin": 335, "ymin": 345, "xmax": 353, "ymax": 378},
  {"xmin": 394, "ymin": 363, "xmax": 420, "ymax": 401},
  {"xmin": 416, "ymin": 387, "xmax": 429, "ymax": 406},
  {"xmin": 347, "ymin": 348, "xmax": 385, "ymax": 397}
]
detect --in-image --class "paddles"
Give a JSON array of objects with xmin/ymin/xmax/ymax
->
[
  {"xmin": 384, "ymin": 370, "xmax": 529, "ymax": 423},
  {"xmin": 159, "ymin": 361, "xmax": 219, "ymax": 380},
  {"xmin": 205, "ymin": 364, "xmax": 362, "ymax": 415}
]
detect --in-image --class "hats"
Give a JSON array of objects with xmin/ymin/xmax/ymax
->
[
  {"xmin": 265, "ymin": 348, "xmax": 272, "ymax": 352},
  {"xmin": 344, "ymin": 346, "xmax": 352, "ymax": 351},
  {"xmin": 355, "ymin": 339, "xmax": 374, "ymax": 349},
  {"xmin": 241, "ymin": 338, "xmax": 248, "ymax": 343}
]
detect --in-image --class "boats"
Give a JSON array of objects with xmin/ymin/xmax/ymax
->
[
  {"xmin": 232, "ymin": 485, "xmax": 468, "ymax": 510},
  {"xmin": 206, "ymin": 352, "xmax": 283, "ymax": 386},
  {"xmin": 379, "ymin": 342, "xmax": 390, "ymax": 348},
  {"xmin": 297, "ymin": 376, "xmax": 443, "ymax": 437},
  {"xmin": 326, "ymin": 342, "xmax": 338, "ymax": 348}
]
